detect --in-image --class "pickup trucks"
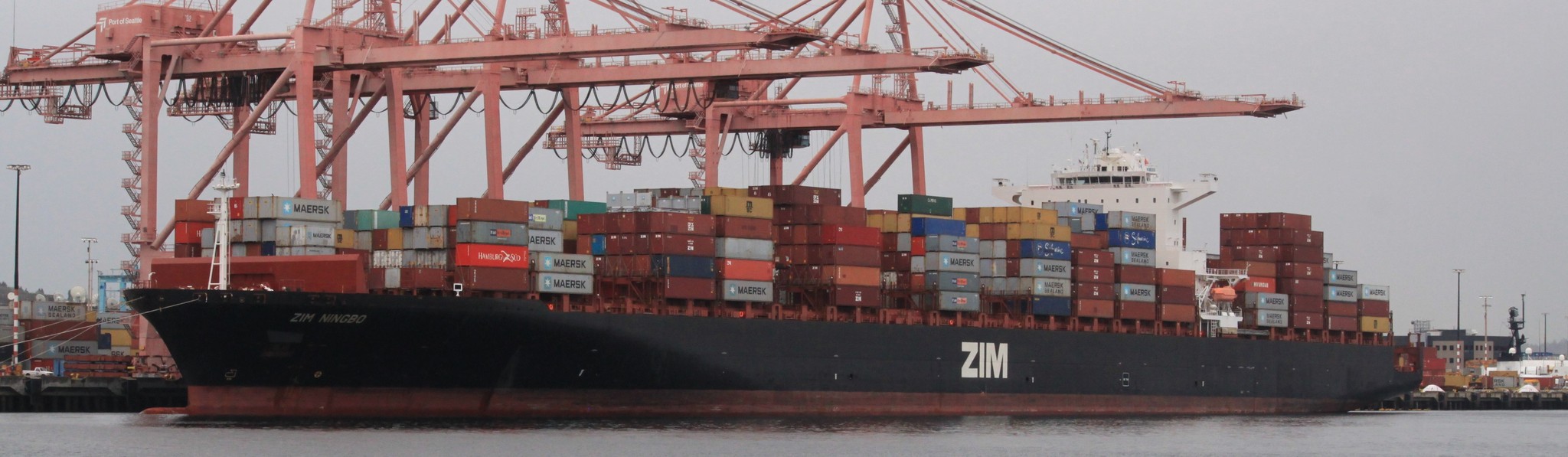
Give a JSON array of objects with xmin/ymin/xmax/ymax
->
[{"xmin": 21, "ymin": 366, "xmax": 54, "ymax": 378}]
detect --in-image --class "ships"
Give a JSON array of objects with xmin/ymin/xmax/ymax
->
[{"xmin": 117, "ymin": 184, "xmax": 1425, "ymax": 416}]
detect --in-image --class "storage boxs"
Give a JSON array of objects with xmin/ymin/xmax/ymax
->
[
  {"xmin": 0, "ymin": 296, "xmax": 133, "ymax": 378},
  {"xmin": 148, "ymin": 179, "xmax": 1396, "ymax": 337}
]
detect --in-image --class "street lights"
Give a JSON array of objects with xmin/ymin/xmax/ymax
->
[
  {"xmin": 1557, "ymin": 353, "xmax": 1566, "ymax": 368},
  {"xmin": 81, "ymin": 237, "xmax": 100, "ymax": 308},
  {"xmin": 1507, "ymin": 347, "xmax": 1533, "ymax": 375},
  {"xmin": 6, "ymin": 164, "xmax": 31, "ymax": 289}
]
